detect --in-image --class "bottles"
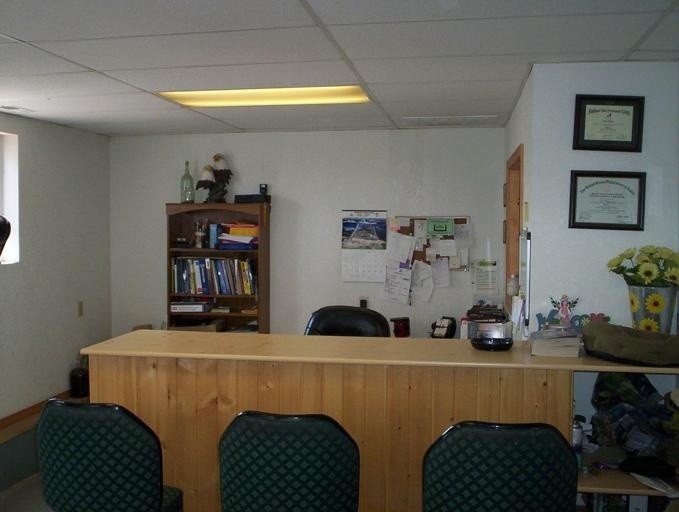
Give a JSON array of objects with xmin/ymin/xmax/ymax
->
[
  {"xmin": 180, "ymin": 158, "xmax": 197, "ymax": 203},
  {"xmin": 571, "ymin": 421, "xmax": 584, "ymax": 473}
]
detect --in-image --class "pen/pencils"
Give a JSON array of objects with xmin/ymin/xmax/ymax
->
[{"xmin": 194, "ymin": 221, "xmax": 207, "ymax": 232}]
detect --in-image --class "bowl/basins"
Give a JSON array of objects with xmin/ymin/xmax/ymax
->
[{"xmin": 474, "ymin": 338, "xmax": 513, "ymax": 351}]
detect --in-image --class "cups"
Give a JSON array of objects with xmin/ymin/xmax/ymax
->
[{"xmin": 192, "ymin": 230, "xmax": 207, "ymax": 249}]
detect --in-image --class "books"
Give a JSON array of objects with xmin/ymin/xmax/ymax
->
[
  {"xmin": 530, "ymin": 327, "xmax": 580, "ymax": 357},
  {"xmin": 169, "ymin": 255, "xmax": 258, "ymax": 313}
]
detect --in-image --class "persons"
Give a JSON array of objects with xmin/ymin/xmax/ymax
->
[{"xmin": 558, "ymin": 300, "xmax": 572, "ymax": 327}]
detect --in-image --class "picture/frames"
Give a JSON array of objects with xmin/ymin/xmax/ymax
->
[
  {"xmin": 572, "ymin": 92, "xmax": 645, "ymax": 152},
  {"xmin": 569, "ymin": 169, "xmax": 647, "ymax": 231}
]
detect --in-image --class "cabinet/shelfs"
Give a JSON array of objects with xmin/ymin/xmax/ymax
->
[
  {"xmin": 164, "ymin": 200, "xmax": 272, "ymax": 333},
  {"xmin": 78, "ymin": 327, "xmax": 678, "ymax": 512}
]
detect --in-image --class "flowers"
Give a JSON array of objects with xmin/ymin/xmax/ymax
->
[{"xmin": 606, "ymin": 242, "xmax": 679, "ymax": 288}]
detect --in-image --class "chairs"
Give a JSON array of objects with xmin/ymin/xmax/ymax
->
[
  {"xmin": 218, "ymin": 410, "xmax": 361, "ymax": 512},
  {"xmin": 421, "ymin": 421, "xmax": 581, "ymax": 512},
  {"xmin": 31, "ymin": 398, "xmax": 186, "ymax": 512},
  {"xmin": 304, "ymin": 304, "xmax": 391, "ymax": 336}
]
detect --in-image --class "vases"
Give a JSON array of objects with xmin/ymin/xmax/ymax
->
[{"xmin": 626, "ymin": 285, "xmax": 678, "ymax": 337}]
